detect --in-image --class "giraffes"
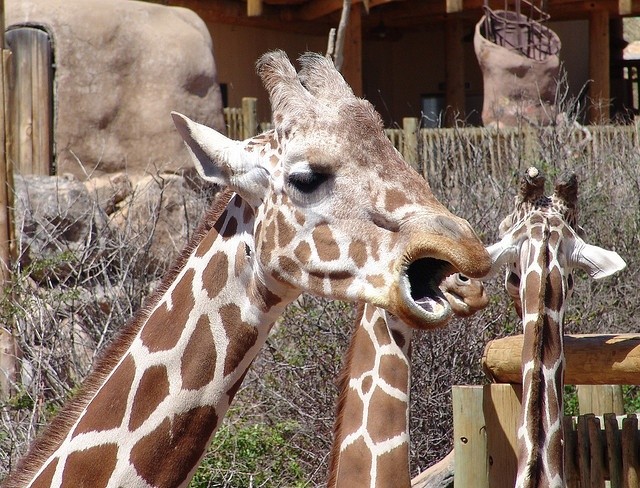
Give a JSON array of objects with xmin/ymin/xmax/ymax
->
[
  {"xmin": 329, "ymin": 301, "xmax": 414, "ymax": 487},
  {"xmin": 486, "ymin": 168, "xmax": 627, "ymax": 488},
  {"xmin": 0, "ymin": 50, "xmax": 492, "ymax": 488}
]
